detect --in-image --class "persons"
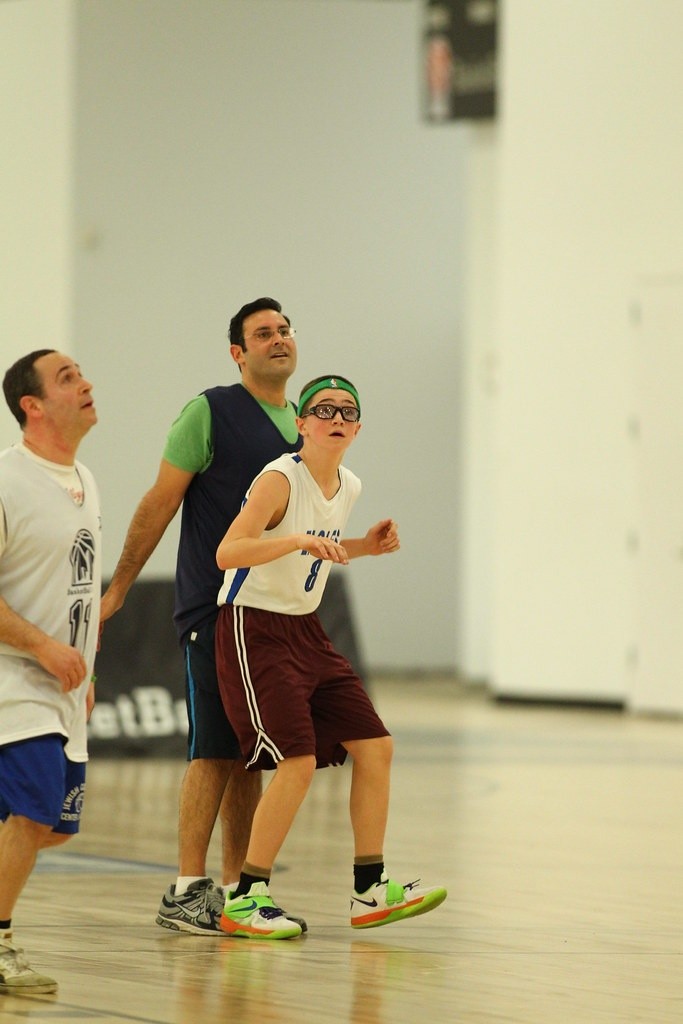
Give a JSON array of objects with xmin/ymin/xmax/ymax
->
[
  {"xmin": 1, "ymin": 349, "xmax": 101, "ymax": 991},
  {"xmin": 214, "ymin": 375, "xmax": 449, "ymax": 942},
  {"xmin": 96, "ymin": 298, "xmax": 306, "ymax": 937}
]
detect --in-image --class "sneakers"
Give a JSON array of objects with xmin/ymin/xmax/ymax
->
[
  {"xmin": 0, "ymin": 927, "xmax": 58, "ymax": 996},
  {"xmin": 217, "ymin": 884, "xmax": 306, "ymax": 935},
  {"xmin": 350, "ymin": 872, "xmax": 446, "ymax": 929},
  {"xmin": 218, "ymin": 881, "xmax": 302, "ymax": 939},
  {"xmin": 155, "ymin": 879, "xmax": 228, "ymax": 936}
]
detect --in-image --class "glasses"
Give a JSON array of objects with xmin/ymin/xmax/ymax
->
[
  {"xmin": 300, "ymin": 404, "xmax": 360, "ymax": 423},
  {"xmin": 241, "ymin": 328, "xmax": 297, "ymax": 342}
]
